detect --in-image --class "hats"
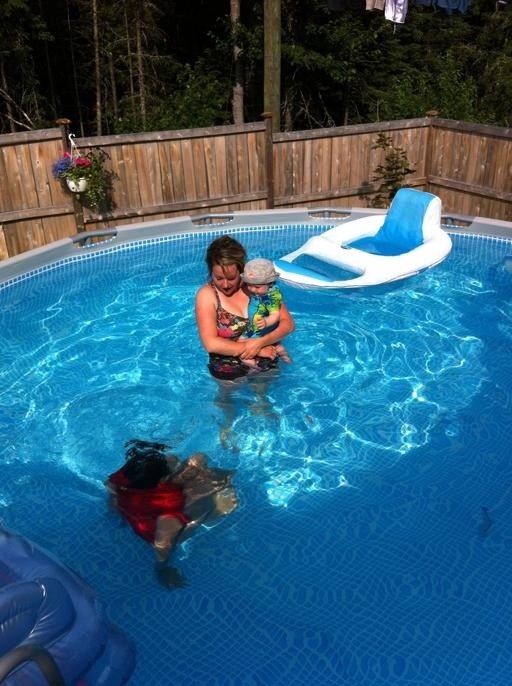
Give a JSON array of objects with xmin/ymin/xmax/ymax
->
[{"xmin": 238, "ymin": 256, "xmax": 281, "ymax": 287}]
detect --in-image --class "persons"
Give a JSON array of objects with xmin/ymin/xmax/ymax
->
[
  {"xmin": 105, "ymin": 439, "xmax": 236, "ymax": 590},
  {"xmin": 239, "ymin": 258, "xmax": 292, "ymax": 369},
  {"xmin": 195, "ymin": 235, "xmax": 295, "ymax": 451}
]
